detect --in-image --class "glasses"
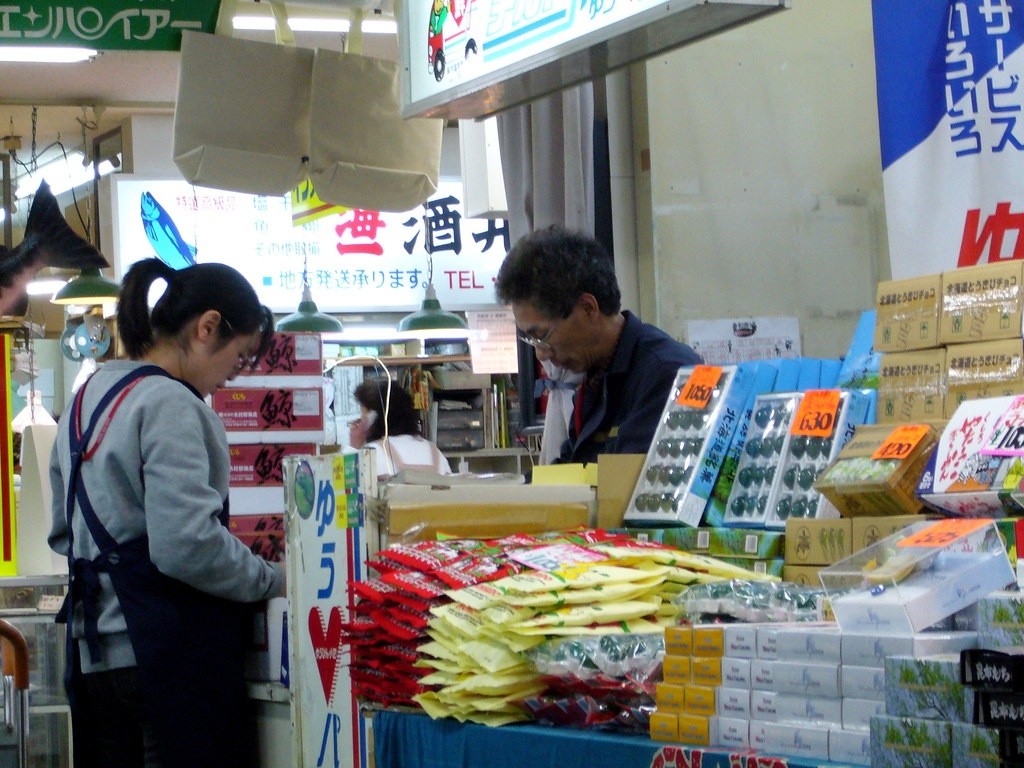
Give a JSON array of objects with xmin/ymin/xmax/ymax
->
[
  {"xmin": 225, "ymin": 319, "xmax": 255, "ymax": 371},
  {"xmin": 519, "ymin": 303, "xmax": 568, "ymax": 350}
]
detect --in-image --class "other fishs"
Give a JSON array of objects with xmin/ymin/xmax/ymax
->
[{"xmin": 0, "ymin": 177, "xmax": 112, "ymax": 333}]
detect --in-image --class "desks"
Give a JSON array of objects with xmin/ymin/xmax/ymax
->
[{"xmin": 369, "ymin": 703, "xmax": 864, "ymax": 767}]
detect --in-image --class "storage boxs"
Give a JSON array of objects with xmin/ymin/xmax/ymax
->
[
  {"xmin": 340, "ymin": 343, "xmax": 406, "ymax": 357},
  {"xmin": 437, "ymin": 409, "xmax": 484, "ymax": 449},
  {"xmin": 84, "ymin": 305, "xmax": 127, "ymax": 361},
  {"xmin": 202, "ymin": 333, "xmax": 323, "ymax": 562},
  {"xmin": 254, "ymin": 597, "xmax": 289, "ymax": 687},
  {"xmin": 621, "ymin": 259, "xmax": 1024, "ymax": 767},
  {"xmin": 431, "ymin": 370, "xmax": 491, "ymax": 390},
  {"xmin": 379, "ymin": 454, "xmax": 648, "ymax": 561}
]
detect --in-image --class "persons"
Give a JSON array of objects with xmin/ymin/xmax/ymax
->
[
  {"xmin": 494, "ymin": 224, "xmax": 706, "ymax": 493},
  {"xmin": 47, "ymin": 259, "xmax": 286, "ymax": 768},
  {"xmin": 347, "ymin": 379, "xmax": 455, "ymax": 481}
]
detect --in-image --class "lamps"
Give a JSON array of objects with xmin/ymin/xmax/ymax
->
[
  {"xmin": 396, "ymin": 198, "xmax": 470, "ymax": 331},
  {"xmin": 51, "ymin": 107, "xmax": 122, "ymax": 306},
  {"xmin": 274, "ymin": 243, "xmax": 342, "ymax": 334},
  {"xmin": 11, "ymin": 391, "xmax": 57, "ymax": 433},
  {"xmin": 232, "ymin": 0, "xmax": 397, "ymax": 34}
]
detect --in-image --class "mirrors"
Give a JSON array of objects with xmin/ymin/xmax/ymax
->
[{"xmin": 93, "ymin": 125, "xmax": 122, "ymax": 279}]
